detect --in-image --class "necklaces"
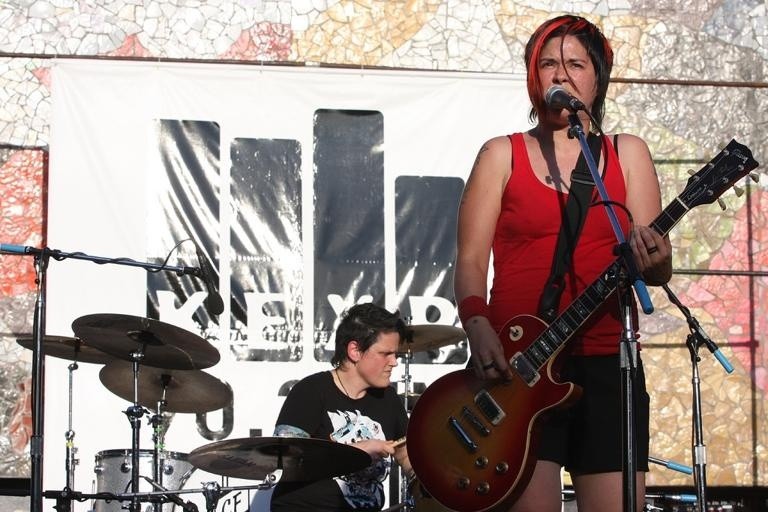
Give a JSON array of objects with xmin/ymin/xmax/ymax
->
[{"xmin": 335, "ymin": 367, "xmax": 349, "ymax": 404}]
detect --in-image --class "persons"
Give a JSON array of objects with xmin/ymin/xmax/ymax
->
[
  {"xmin": 270, "ymin": 303, "xmax": 418, "ymax": 512},
  {"xmin": 454, "ymin": 15, "xmax": 672, "ymax": 512}
]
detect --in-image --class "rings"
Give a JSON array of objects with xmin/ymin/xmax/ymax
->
[
  {"xmin": 484, "ymin": 360, "xmax": 497, "ymax": 369},
  {"xmin": 647, "ymin": 247, "xmax": 658, "ymax": 254}
]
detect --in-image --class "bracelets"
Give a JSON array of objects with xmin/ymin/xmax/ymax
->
[{"xmin": 458, "ymin": 296, "xmax": 493, "ymax": 332}]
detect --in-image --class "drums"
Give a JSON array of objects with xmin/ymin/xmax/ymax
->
[
  {"xmin": 93, "ymin": 448, "xmax": 194, "ymax": 512},
  {"xmin": 173, "ymin": 467, "xmax": 285, "ymax": 511},
  {"xmin": 402, "ymin": 468, "xmax": 449, "ymax": 512}
]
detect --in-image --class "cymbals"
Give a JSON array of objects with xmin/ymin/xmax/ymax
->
[
  {"xmin": 185, "ymin": 436, "xmax": 372, "ymax": 481},
  {"xmin": 397, "ymin": 324, "xmax": 467, "ymax": 356},
  {"xmin": 17, "ymin": 335, "xmax": 118, "ymax": 364},
  {"xmin": 72, "ymin": 313, "xmax": 220, "ymax": 370},
  {"xmin": 98, "ymin": 359, "xmax": 232, "ymax": 413}
]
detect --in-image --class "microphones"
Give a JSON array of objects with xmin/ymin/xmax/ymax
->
[
  {"xmin": 546, "ymin": 86, "xmax": 585, "ymax": 111},
  {"xmin": 145, "ymin": 477, "xmax": 199, "ymax": 512},
  {"xmin": 196, "ymin": 248, "xmax": 224, "ymax": 315}
]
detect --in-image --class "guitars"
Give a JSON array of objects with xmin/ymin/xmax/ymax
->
[{"xmin": 406, "ymin": 139, "xmax": 759, "ymax": 512}]
